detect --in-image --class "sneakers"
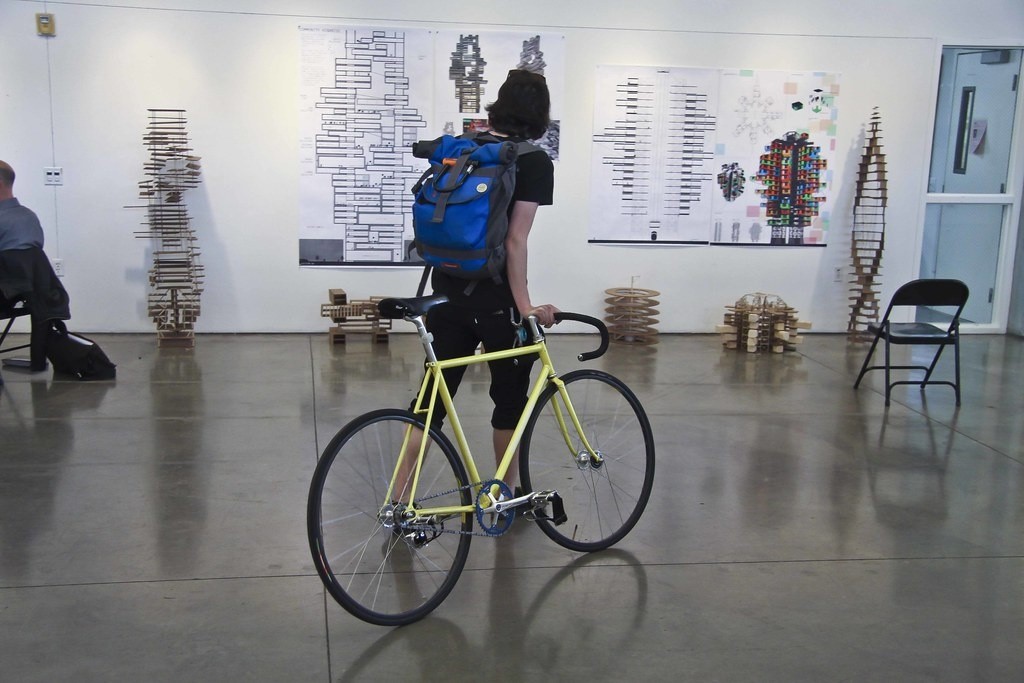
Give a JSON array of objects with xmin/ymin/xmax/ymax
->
[{"xmin": 488, "ymin": 485, "xmax": 546, "ymax": 519}]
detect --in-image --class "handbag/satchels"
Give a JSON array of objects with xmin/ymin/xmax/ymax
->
[{"xmin": 46, "ymin": 319, "xmax": 116, "ymax": 370}]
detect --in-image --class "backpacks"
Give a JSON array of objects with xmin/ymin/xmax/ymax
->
[{"xmin": 407, "ymin": 126, "xmax": 546, "ymax": 298}]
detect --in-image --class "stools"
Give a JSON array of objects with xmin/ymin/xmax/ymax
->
[{"xmin": 1, "ymin": 294, "xmax": 53, "ymax": 373}]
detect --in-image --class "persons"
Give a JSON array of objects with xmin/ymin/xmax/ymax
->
[
  {"xmin": 391, "ymin": 69, "xmax": 562, "ymax": 535},
  {"xmin": 0, "ymin": 161, "xmax": 43, "ymax": 250}
]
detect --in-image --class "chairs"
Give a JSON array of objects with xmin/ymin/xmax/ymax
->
[{"xmin": 852, "ymin": 277, "xmax": 970, "ymax": 407}]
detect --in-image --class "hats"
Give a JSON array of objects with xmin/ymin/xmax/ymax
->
[{"xmin": 492, "ymin": 70, "xmax": 549, "ymax": 122}]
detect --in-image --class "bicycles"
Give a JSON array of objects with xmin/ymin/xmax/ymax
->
[{"xmin": 302, "ymin": 291, "xmax": 659, "ymax": 631}]
